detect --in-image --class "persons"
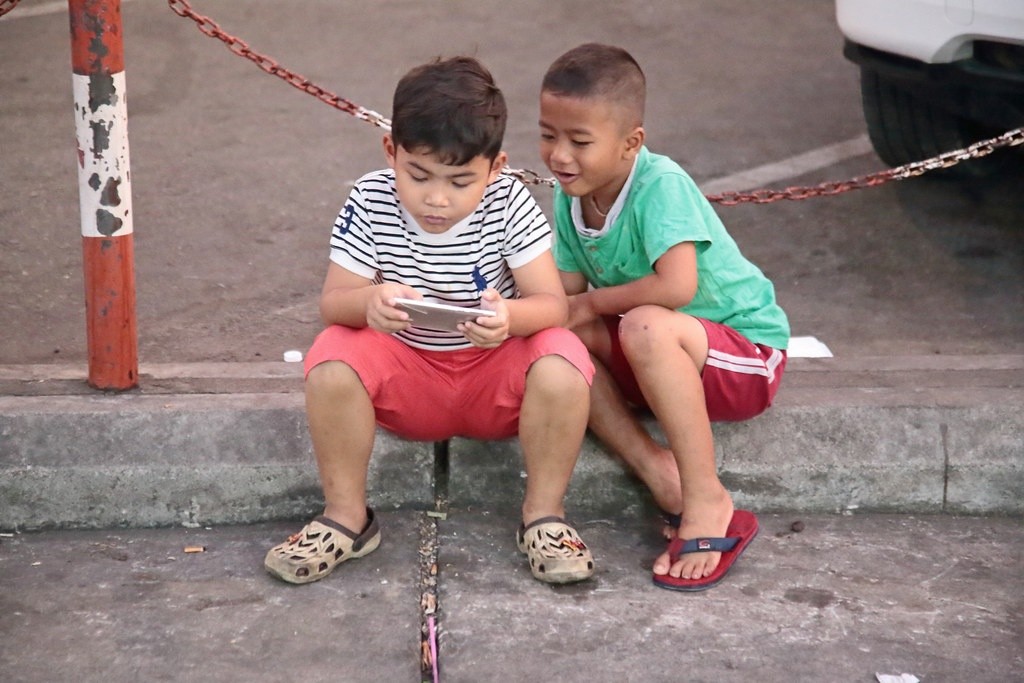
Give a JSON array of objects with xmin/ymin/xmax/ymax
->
[
  {"xmin": 537, "ymin": 42, "xmax": 790, "ymax": 592},
  {"xmin": 266, "ymin": 57, "xmax": 595, "ymax": 587}
]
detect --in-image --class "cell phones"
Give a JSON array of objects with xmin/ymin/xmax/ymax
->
[{"xmin": 390, "ymin": 297, "xmax": 498, "ymax": 335}]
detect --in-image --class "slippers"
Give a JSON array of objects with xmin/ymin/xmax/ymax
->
[
  {"xmin": 660, "ymin": 510, "xmax": 683, "ymax": 540},
  {"xmin": 652, "ymin": 510, "xmax": 760, "ymax": 592}
]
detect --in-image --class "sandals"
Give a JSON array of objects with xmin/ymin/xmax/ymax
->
[
  {"xmin": 516, "ymin": 515, "xmax": 595, "ymax": 584},
  {"xmin": 264, "ymin": 506, "xmax": 382, "ymax": 585}
]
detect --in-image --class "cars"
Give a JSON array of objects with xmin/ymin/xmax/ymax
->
[{"xmin": 833, "ymin": 0, "xmax": 1024, "ymax": 180}]
